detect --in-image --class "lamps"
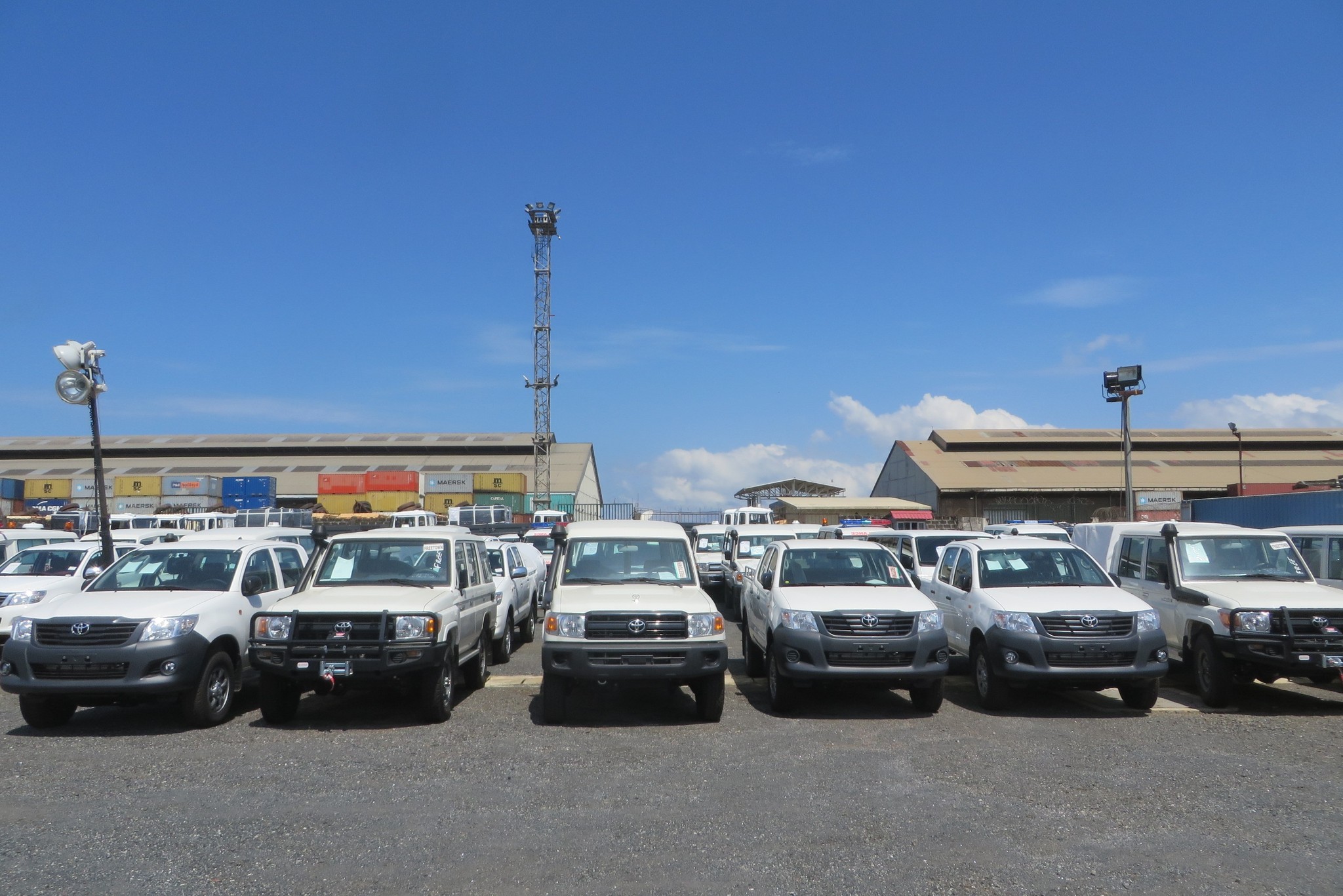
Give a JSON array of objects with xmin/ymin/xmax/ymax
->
[{"xmin": 970, "ymin": 494, "xmax": 974, "ymax": 500}]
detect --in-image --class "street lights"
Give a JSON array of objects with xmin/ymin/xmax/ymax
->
[
  {"xmin": 1103, "ymin": 364, "xmax": 1142, "ymax": 518},
  {"xmin": 1228, "ymin": 422, "xmax": 1243, "ymax": 496},
  {"xmin": 53, "ymin": 339, "xmax": 120, "ymax": 585}
]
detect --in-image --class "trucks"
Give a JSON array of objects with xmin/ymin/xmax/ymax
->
[{"xmin": 21, "ymin": 501, "xmax": 573, "ymax": 530}]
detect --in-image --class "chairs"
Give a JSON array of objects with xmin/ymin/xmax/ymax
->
[
  {"xmin": 202, "ymin": 562, "xmax": 226, "ymax": 575},
  {"xmin": 161, "ymin": 557, "xmax": 195, "ymax": 588},
  {"xmin": 46, "ymin": 557, "xmax": 65, "ymax": 573},
  {"xmin": 488, "ymin": 554, "xmax": 502, "ymax": 572},
  {"xmin": 426, "ymin": 556, "xmax": 441, "ymax": 568},
  {"xmin": 785, "ymin": 560, "xmax": 807, "ymax": 583},
  {"xmin": 576, "ymin": 558, "xmax": 604, "ymax": 576}
]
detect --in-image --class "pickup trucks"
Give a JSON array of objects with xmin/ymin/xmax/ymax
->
[
  {"xmin": 1073, "ymin": 519, "xmax": 1342, "ymax": 704},
  {"xmin": 741, "ymin": 539, "xmax": 949, "ymax": 716},
  {"xmin": 918, "ymin": 536, "xmax": 1168, "ymax": 711},
  {"xmin": 485, "ymin": 541, "xmax": 538, "ymax": 660}
]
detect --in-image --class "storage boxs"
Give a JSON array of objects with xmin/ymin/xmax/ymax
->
[
  {"xmin": 0, "ymin": 476, "xmax": 277, "ymax": 515},
  {"xmin": 1133, "ymin": 482, "xmax": 1343, "ymax": 529},
  {"xmin": 317, "ymin": 471, "xmax": 573, "ymax": 523}
]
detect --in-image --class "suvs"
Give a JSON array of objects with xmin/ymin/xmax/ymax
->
[
  {"xmin": 441, "ymin": 523, "xmax": 558, "ymax": 602},
  {"xmin": 0, "ymin": 527, "xmax": 314, "ymax": 664},
  {"xmin": 693, "ymin": 504, "xmax": 1070, "ymax": 595},
  {"xmin": 538, "ymin": 518, "xmax": 727, "ymax": 722},
  {"xmin": 0, "ymin": 540, "xmax": 308, "ymax": 729},
  {"xmin": 248, "ymin": 527, "xmax": 495, "ymax": 718}
]
